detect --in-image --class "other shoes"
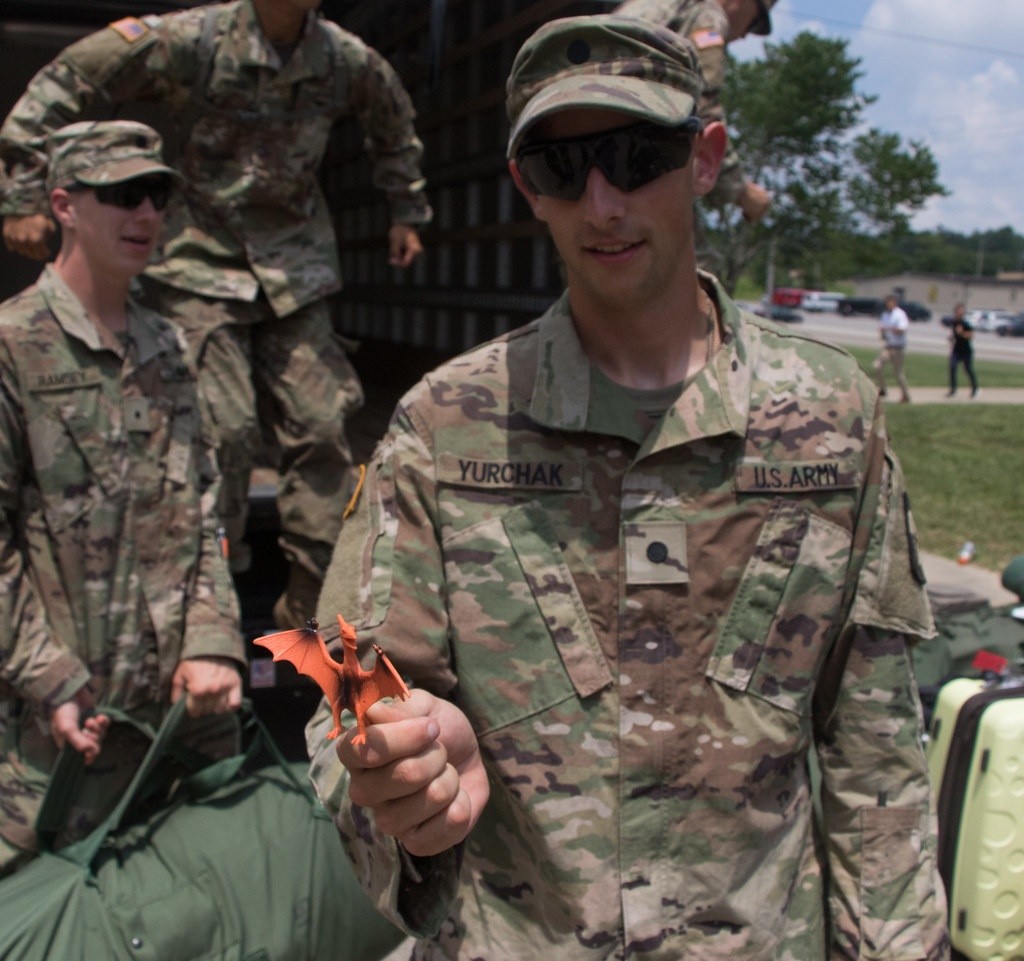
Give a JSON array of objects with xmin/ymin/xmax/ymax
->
[
  {"xmin": 878, "ymin": 390, "xmax": 885, "ymax": 396},
  {"xmin": 901, "ymin": 397, "xmax": 911, "ymax": 402},
  {"xmin": 971, "ymin": 384, "xmax": 978, "ymax": 395},
  {"xmin": 949, "ymin": 387, "xmax": 958, "ymax": 397}
]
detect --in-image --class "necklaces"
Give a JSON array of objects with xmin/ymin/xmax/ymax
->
[{"xmin": 705, "ymin": 295, "xmax": 715, "ymax": 363}]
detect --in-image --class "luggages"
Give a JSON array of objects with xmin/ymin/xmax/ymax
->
[{"xmin": 927, "ymin": 670, "xmax": 1024, "ymax": 961}]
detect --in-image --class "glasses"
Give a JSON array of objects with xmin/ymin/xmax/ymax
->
[
  {"xmin": 63, "ymin": 174, "xmax": 172, "ymax": 212},
  {"xmin": 746, "ymin": 0, "xmax": 771, "ymax": 35},
  {"xmin": 514, "ymin": 115, "xmax": 706, "ymax": 200}
]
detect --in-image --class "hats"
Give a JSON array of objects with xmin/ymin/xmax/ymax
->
[
  {"xmin": 46, "ymin": 120, "xmax": 187, "ymax": 203},
  {"xmin": 505, "ymin": 14, "xmax": 706, "ymax": 159}
]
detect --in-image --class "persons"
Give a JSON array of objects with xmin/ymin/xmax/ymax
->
[
  {"xmin": 613, "ymin": 0, "xmax": 779, "ymax": 273},
  {"xmin": 0, "ymin": 121, "xmax": 247, "ymax": 873},
  {"xmin": 0, "ymin": 0, "xmax": 433, "ymax": 723},
  {"xmin": 871, "ymin": 294, "xmax": 912, "ymax": 404},
  {"xmin": 942, "ymin": 302, "xmax": 980, "ymax": 398},
  {"xmin": 305, "ymin": 14, "xmax": 949, "ymax": 961}
]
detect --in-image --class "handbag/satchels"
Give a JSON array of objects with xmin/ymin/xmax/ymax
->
[
  {"xmin": 912, "ymin": 600, "xmax": 1024, "ymax": 696},
  {"xmin": 0, "ymin": 691, "xmax": 408, "ymax": 961}
]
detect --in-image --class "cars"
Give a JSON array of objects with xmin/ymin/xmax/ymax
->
[
  {"xmin": 942, "ymin": 309, "xmax": 1024, "ymax": 336},
  {"xmin": 736, "ymin": 289, "xmax": 932, "ymax": 324}
]
